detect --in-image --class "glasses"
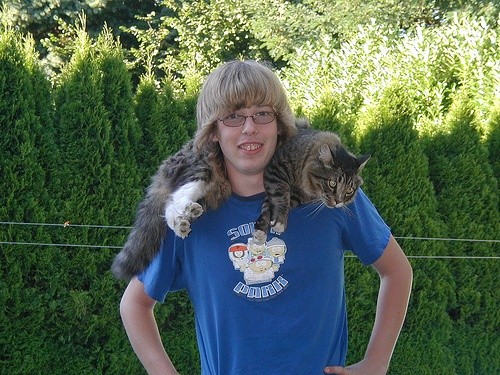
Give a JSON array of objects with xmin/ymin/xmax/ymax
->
[{"xmin": 216, "ymin": 105, "xmax": 278, "ymax": 127}]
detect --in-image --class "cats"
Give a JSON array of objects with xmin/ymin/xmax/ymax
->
[{"xmin": 110, "ymin": 117, "xmax": 372, "ymax": 287}]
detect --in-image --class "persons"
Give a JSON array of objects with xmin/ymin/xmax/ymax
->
[{"xmin": 117, "ymin": 58, "xmax": 416, "ymax": 375}]
